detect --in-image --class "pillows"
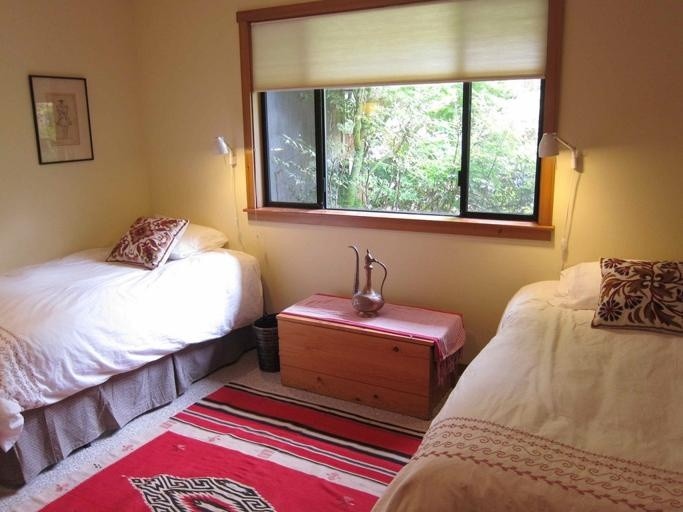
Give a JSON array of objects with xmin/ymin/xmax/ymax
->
[
  {"xmin": 170, "ymin": 223, "xmax": 228, "ymax": 260},
  {"xmin": 555, "ymin": 261, "xmax": 601, "ymax": 310},
  {"xmin": 590, "ymin": 258, "xmax": 683, "ymax": 336},
  {"xmin": 105, "ymin": 216, "xmax": 190, "ymax": 270}
]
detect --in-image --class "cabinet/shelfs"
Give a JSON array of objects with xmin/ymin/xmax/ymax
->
[{"xmin": 276, "ymin": 291, "xmax": 464, "ymax": 422}]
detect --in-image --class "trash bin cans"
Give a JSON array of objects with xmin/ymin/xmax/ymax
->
[{"xmin": 252, "ymin": 313, "xmax": 281, "ymax": 373}]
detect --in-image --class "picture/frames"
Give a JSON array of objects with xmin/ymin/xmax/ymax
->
[{"xmin": 28, "ymin": 74, "xmax": 95, "ymax": 166}]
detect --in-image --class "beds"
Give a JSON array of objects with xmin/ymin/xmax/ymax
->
[
  {"xmin": 0, "ymin": 247, "xmax": 264, "ymax": 489},
  {"xmin": 371, "ymin": 281, "xmax": 683, "ymax": 512}
]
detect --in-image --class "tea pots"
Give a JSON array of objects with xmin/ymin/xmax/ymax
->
[{"xmin": 347, "ymin": 242, "xmax": 388, "ymax": 318}]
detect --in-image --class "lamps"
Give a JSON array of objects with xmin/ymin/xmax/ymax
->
[
  {"xmin": 538, "ymin": 131, "xmax": 581, "ymax": 273},
  {"xmin": 212, "ymin": 136, "xmax": 239, "ymax": 244}
]
